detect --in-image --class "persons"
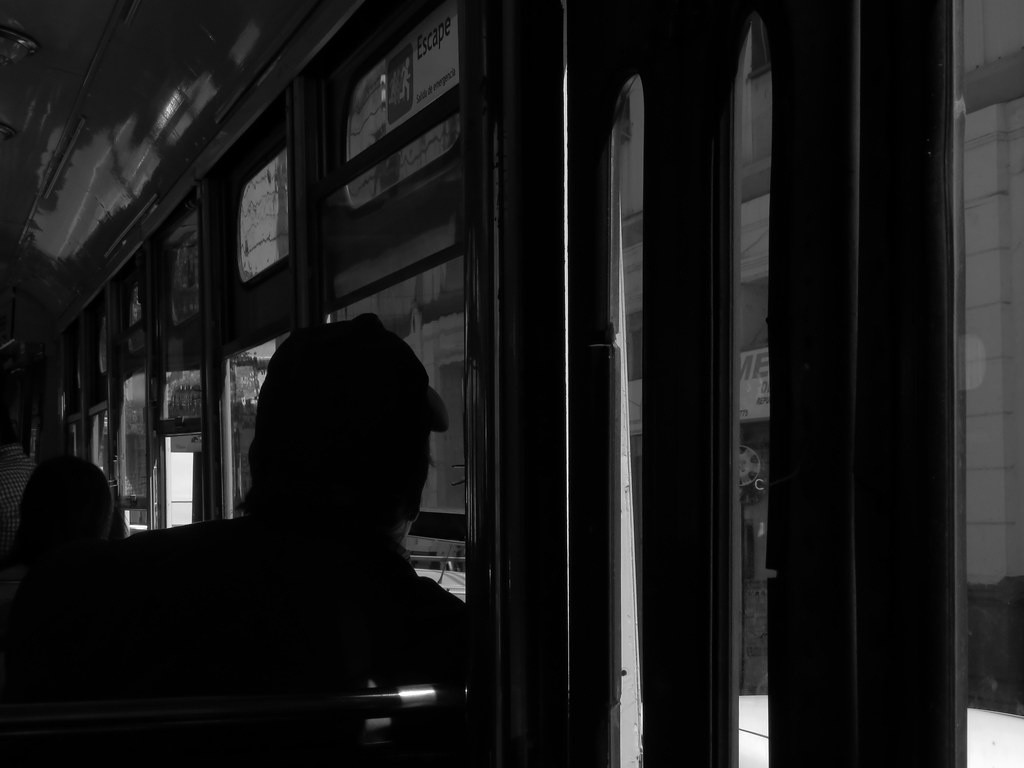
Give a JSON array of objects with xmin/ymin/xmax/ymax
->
[
  {"xmin": 0, "ymin": 398, "xmax": 39, "ymax": 558},
  {"xmin": 0, "ymin": 317, "xmax": 465, "ymax": 704},
  {"xmin": 0, "ymin": 453, "xmax": 114, "ymax": 654}
]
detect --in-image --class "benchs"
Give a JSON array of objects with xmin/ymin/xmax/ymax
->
[{"xmin": 0, "ymin": 683, "xmax": 472, "ymax": 768}]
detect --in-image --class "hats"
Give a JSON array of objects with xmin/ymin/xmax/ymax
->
[{"xmin": 267, "ymin": 312, "xmax": 429, "ymax": 397}]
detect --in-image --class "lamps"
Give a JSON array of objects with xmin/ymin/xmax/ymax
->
[{"xmin": 0, "ymin": 28, "xmax": 40, "ymax": 66}]
